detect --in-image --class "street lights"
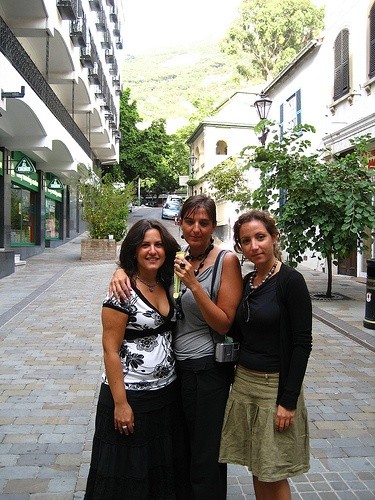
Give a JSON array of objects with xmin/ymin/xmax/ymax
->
[{"xmin": 252, "ymin": 90, "xmax": 274, "ymax": 147}]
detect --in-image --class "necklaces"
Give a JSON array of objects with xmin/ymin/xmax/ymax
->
[
  {"xmin": 136, "ymin": 274, "xmax": 159, "ymax": 292},
  {"xmin": 176, "ymin": 239, "xmax": 214, "ymax": 320},
  {"xmin": 249, "ymin": 259, "xmax": 278, "ymax": 290}
]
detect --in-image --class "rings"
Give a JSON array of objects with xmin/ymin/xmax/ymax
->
[
  {"xmin": 179, "ymin": 263, "xmax": 185, "ymax": 267},
  {"xmin": 122, "ymin": 426, "xmax": 127, "ymax": 428}
]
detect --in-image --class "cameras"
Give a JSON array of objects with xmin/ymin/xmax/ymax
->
[{"xmin": 215, "ymin": 343, "xmax": 240, "ymax": 362}]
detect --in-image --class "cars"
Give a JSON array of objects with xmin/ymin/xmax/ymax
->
[{"xmin": 162, "ymin": 195, "xmax": 184, "ymax": 220}]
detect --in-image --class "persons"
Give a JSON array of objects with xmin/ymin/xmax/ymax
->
[
  {"xmin": 109, "ymin": 195, "xmax": 242, "ymax": 500},
  {"xmin": 84, "ymin": 219, "xmax": 182, "ymax": 500},
  {"xmin": 218, "ymin": 211, "xmax": 312, "ymax": 500}
]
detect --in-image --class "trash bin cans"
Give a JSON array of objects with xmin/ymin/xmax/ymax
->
[{"xmin": 363, "ymin": 259, "xmax": 375, "ymax": 329}]
